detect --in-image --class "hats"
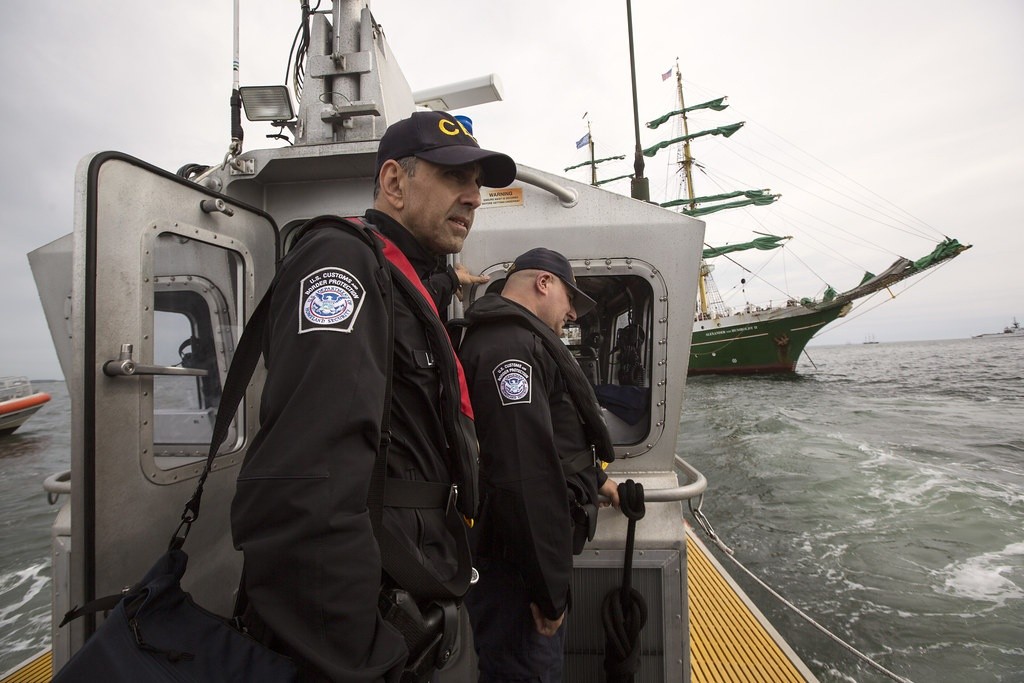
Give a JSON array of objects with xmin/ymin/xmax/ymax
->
[
  {"xmin": 375, "ymin": 110, "xmax": 516, "ymax": 189},
  {"xmin": 505, "ymin": 248, "xmax": 596, "ymax": 318}
]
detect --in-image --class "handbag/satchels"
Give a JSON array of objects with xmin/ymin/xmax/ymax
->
[{"xmin": 47, "ymin": 518, "xmax": 325, "ymax": 683}]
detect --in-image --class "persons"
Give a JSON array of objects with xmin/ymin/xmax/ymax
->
[
  {"xmin": 224, "ymin": 110, "xmax": 519, "ymax": 682},
  {"xmin": 455, "ymin": 248, "xmax": 620, "ymax": 682}
]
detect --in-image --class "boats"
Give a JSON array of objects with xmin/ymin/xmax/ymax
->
[
  {"xmin": 974, "ymin": 317, "xmax": 1024, "ymax": 339},
  {"xmin": 0, "ymin": 375, "xmax": 53, "ymax": 438}
]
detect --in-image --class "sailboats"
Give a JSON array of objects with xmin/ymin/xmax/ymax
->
[{"xmin": 563, "ymin": 0, "xmax": 973, "ymax": 380}]
